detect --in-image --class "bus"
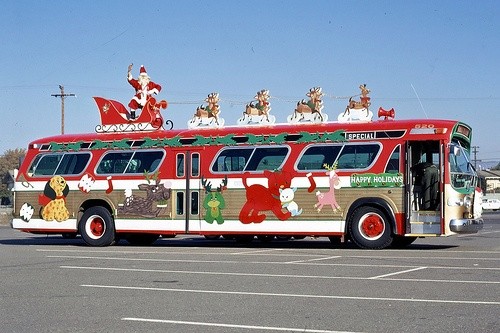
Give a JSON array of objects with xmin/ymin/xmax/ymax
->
[{"xmin": 12, "ymin": 63, "xmax": 484, "ymax": 251}]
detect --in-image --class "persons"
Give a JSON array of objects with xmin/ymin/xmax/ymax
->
[
  {"xmin": 410, "ymin": 162, "xmax": 439, "ymax": 212},
  {"xmin": 124, "ymin": 63, "xmax": 162, "ymax": 121}
]
